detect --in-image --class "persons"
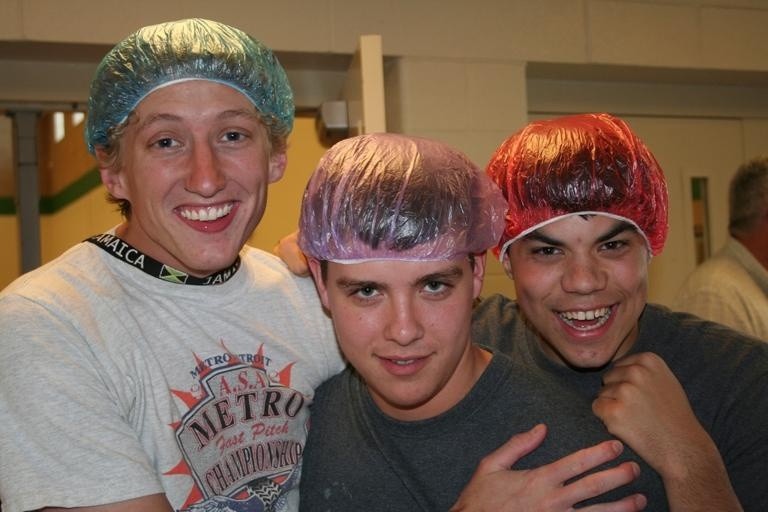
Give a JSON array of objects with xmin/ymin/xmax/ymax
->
[
  {"xmin": 300, "ymin": 132, "xmax": 672, "ymax": 510},
  {"xmin": 0, "ymin": 17, "xmax": 649, "ymax": 511},
  {"xmin": 272, "ymin": 111, "xmax": 766, "ymax": 511},
  {"xmin": 666, "ymin": 154, "xmax": 767, "ymax": 341}
]
